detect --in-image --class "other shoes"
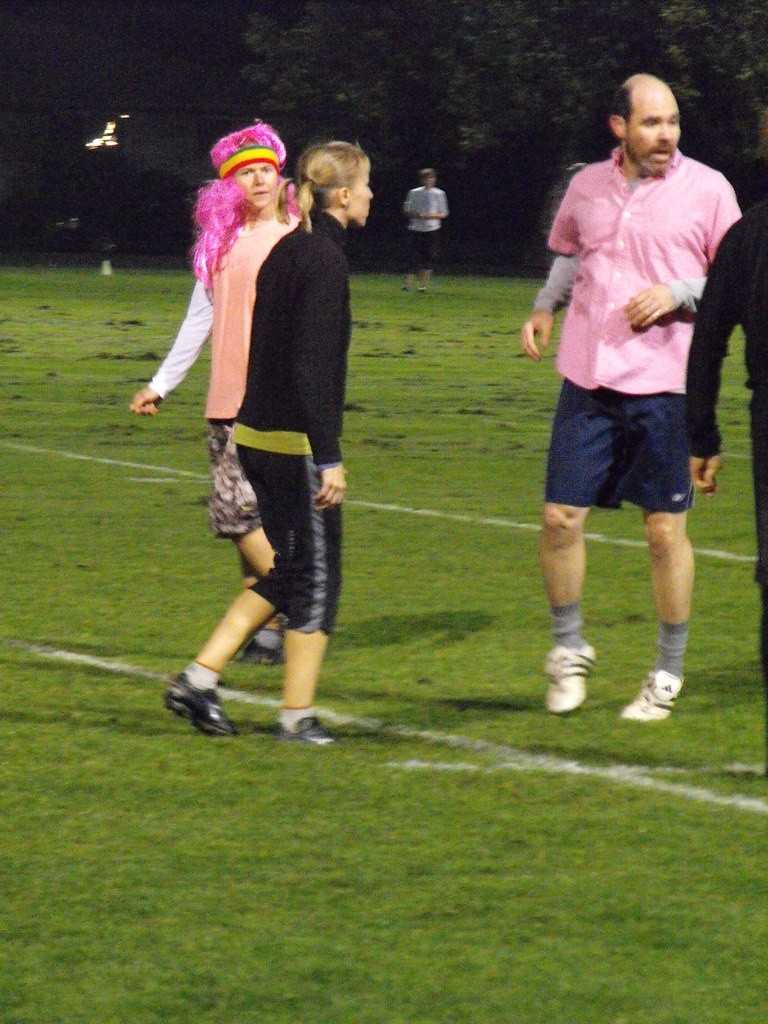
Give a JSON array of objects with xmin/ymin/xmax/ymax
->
[{"xmin": 235, "ymin": 635, "xmax": 285, "ymax": 666}]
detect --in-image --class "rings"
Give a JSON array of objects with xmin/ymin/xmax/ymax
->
[{"xmin": 656, "ymin": 313, "xmax": 659, "ymax": 319}]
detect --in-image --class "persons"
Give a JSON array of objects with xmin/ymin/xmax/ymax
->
[
  {"xmin": 166, "ymin": 142, "xmax": 374, "ymax": 745},
  {"xmin": 403, "ymin": 168, "xmax": 450, "ymax": 291},
  {"xmin": 131, "ymin": 123, "xmax": 300, "ymax": 665},
  {"xmin": 686, "ymin": 198, "xmax": 768, "ymax": 778},
  {"xmin": 518, "ymin": 73, "xmax": 743, "ymax": 720}
]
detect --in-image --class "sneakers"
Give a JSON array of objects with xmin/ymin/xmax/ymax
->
[
  {"xmin": 164, "ymin": 671, "xmax": 239, "ymax": 737},
  {"xmin": 620, "ymin": 669, "xmax": 684, "ymax": 721},
  {"xmin": 274, "ymin": 717, "xmax": 335, "ymax": 747},
  {"xmin": 543, "ymin": 642, "xmax": 596, "ymax": 712}
]
